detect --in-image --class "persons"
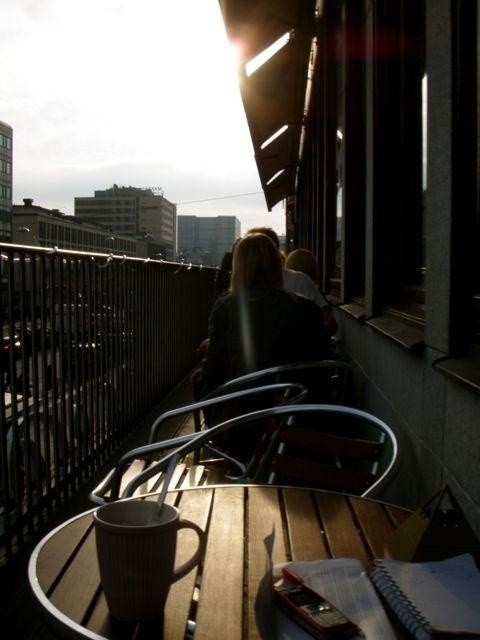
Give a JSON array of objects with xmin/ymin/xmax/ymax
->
[{"xmin": 184, "ymin": 230, "xmax": 337, "ymax": 479}]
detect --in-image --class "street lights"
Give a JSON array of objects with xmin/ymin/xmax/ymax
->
[
  {"xmin": 104, "ymin": 236, "xmax": 115, "ymax": 257},
  {"xmin": 17, "ymin": 225, "xmax": 40, "ymax": 246}
]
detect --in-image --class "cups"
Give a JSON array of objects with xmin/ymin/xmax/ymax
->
[{"xmin": 93, "ymin": 500, "xmax": 207, "ymax": 622}]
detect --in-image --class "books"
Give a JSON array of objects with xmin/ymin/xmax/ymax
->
[{"xmin": 368, "ymin": 553, "xmax": 480, "ymax": 640}]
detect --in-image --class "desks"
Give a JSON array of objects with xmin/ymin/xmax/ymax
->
[{"xmin": 26, "ymin": 483, "xmax": 432, "ymax": 640}]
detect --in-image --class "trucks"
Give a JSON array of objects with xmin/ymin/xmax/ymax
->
[{"xmin": 53, "ymin": 303, "xmax": 123, "ymax": 345}]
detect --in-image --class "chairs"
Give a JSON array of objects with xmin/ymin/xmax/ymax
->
[{"xmin": 89, "ymin": 337, "xmax": 403, "ymax": 505}]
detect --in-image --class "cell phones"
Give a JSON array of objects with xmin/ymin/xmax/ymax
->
[{"xmin": 273, "ymin": 585, "xmax": 356, "ymax": 640}]
